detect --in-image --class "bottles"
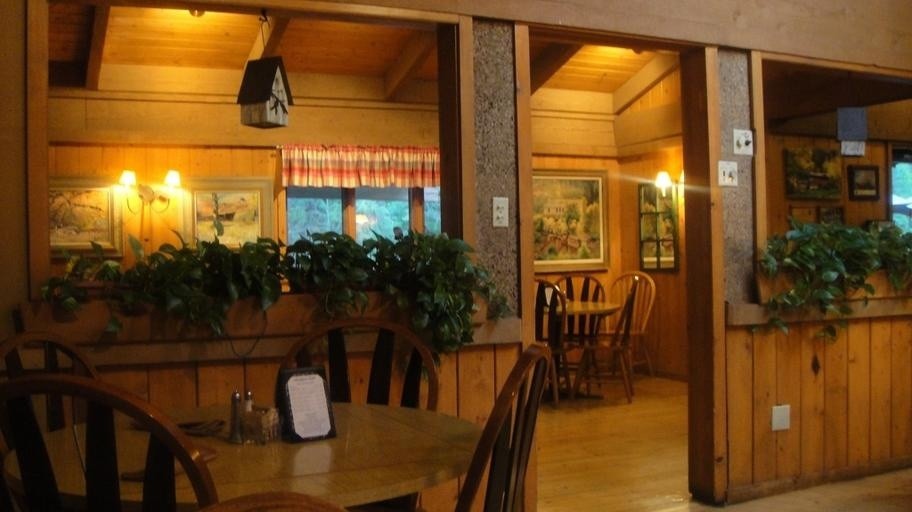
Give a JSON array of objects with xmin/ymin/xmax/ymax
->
[{"xmin": 229, "ymin": 385, "xmax": 256, "ymax": 446}]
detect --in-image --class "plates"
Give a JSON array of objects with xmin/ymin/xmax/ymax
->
[{"xmin": 178, "ymin": 420, "xmax": 226, "ymax": 438}]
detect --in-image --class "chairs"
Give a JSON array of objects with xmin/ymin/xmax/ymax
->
[{"xmin": 529, "ymin": 271, "xmax": 658, "ymax": 409}]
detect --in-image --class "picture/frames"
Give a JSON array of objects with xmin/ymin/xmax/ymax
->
[
  {"xmin": 41, "ymin": 172, "xmax": 275, "ymax": 263},
  {"xmin": 531, "ymin": 167, "xmax": 613, "ymax": 275},
  {"xmin": 846, "ymin": 161, "xmax": 881, "ymax": 203}
]
